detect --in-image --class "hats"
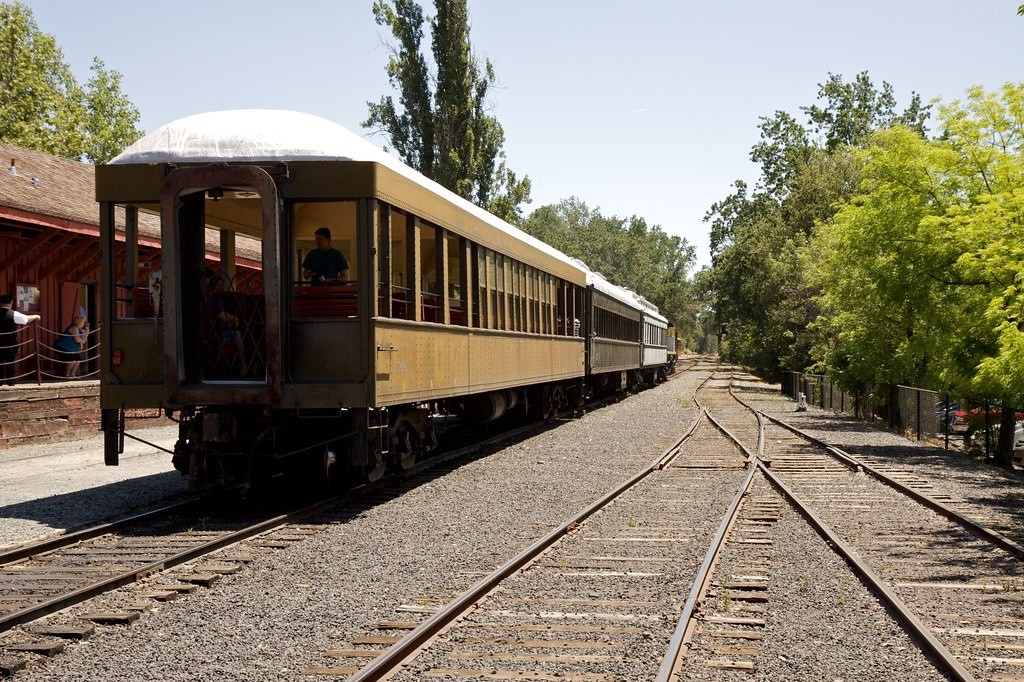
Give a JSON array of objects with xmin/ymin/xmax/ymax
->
[{"xmin": 0, "ymin": 295, "xmax": 13, "ymax": 303}]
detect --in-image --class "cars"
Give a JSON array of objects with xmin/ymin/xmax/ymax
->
[{"xmin": 932, "ymin": 398, "xmax": 1024, "ymax": 458}]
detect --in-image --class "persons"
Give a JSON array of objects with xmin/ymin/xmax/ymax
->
[
  {"xmin": 0, "ymin": 295, "xmax": 40, "ymax": 387},
  {"xmin": 207, "ymin": 276, "xmax": 248, "ymax": 372},
  {"xmin": 301, "ymin": 227, "xmax": 346, "ymax": 299},
  {"xmin": 53, "ymin": 316, "xmax": 90, "ymax": 379}
]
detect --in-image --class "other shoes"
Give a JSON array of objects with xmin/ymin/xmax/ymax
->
[{"xmin": 240, "ymin": 370, "xmax": 256, "ymax": 379}]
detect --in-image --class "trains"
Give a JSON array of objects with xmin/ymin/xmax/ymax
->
[{"xmin": 92, "ymin": 106, "xmax": 681, "ymax": 492}]
